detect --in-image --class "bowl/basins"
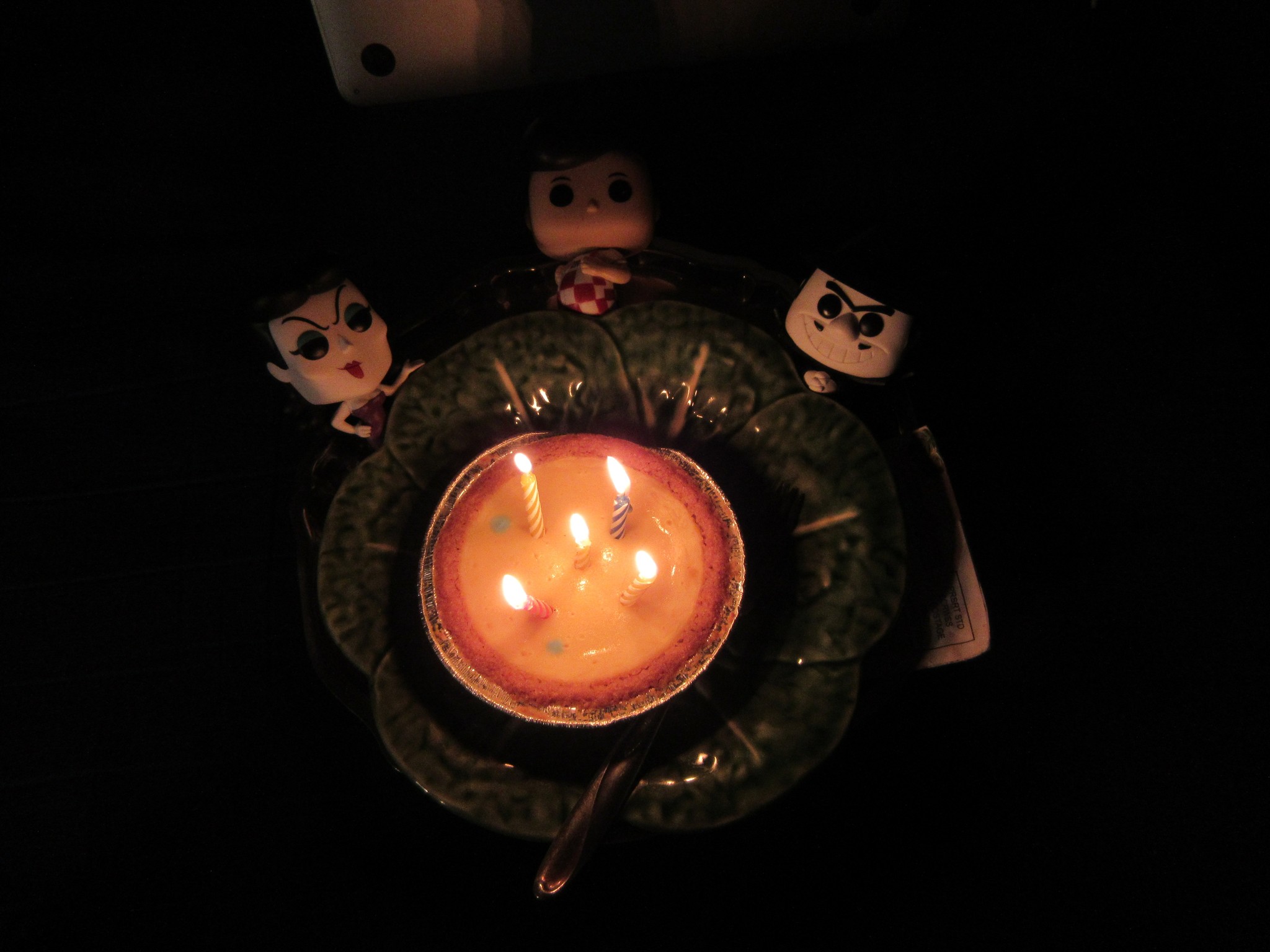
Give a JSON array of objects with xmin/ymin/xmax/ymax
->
[{"xmin": 317, "ymin": 300, "xmax": 908, "ymax": 840}]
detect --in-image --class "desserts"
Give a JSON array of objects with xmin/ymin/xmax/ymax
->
[{"xmin": 420, "ymin": 431, "xmax": 746, "ymax": 727}]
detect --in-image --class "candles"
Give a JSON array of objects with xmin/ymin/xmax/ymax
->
[
  {"xmin": 501, "ymin": 574, "xmax": 553, "ymax": 619},
  {"xmin": 513, "ymin": 453, "xmax": 545, "ymax": 538},
  {"xmin": 569, "ymin": 513, "xmax": 592, "ymax": 570},
  {"xmin": 606, "ymin": 456, "xmax": 632, "ymax": 539},
  {"xmin": 621, "ymin": 551, "xmax": 657, "ymax": 606}
]
